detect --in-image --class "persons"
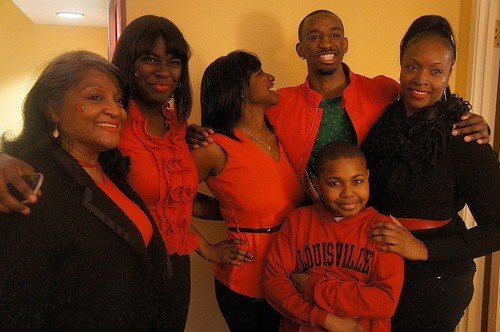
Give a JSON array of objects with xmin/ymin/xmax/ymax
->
[
  {"xmin": 186, "ymin": 10, "xmax": 492, "ymax": 220},
  {"xmin": 261, "ymin": 139, "xmax": 404, "ymax": 332},
  {"xmin": 185, "ymin": 49, "xmax": 308, "ymax": 332},
  {"xmin": 0, "ymin": 15, "xmax": 225, "ymax": 332},
  {"xmin": 359, "ymin": 15, "xmax": 500, "ymax": 332},
  {"xmin": 0, "ymin": 50, "xmax": 173, "ymax": 332}
]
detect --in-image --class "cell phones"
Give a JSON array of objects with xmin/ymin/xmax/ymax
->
[{"xmin": 6, "ymin": 173, "xmax": 44, "ymax": 204}]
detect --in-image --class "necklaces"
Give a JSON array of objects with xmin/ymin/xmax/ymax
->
[
  {"xmin": 79, "ymin": 161, "xmax": 98, "ymax": 167},
  {"xmin": 238, "ymin": 123, "xmax": 271, "ymax": 151}
]
